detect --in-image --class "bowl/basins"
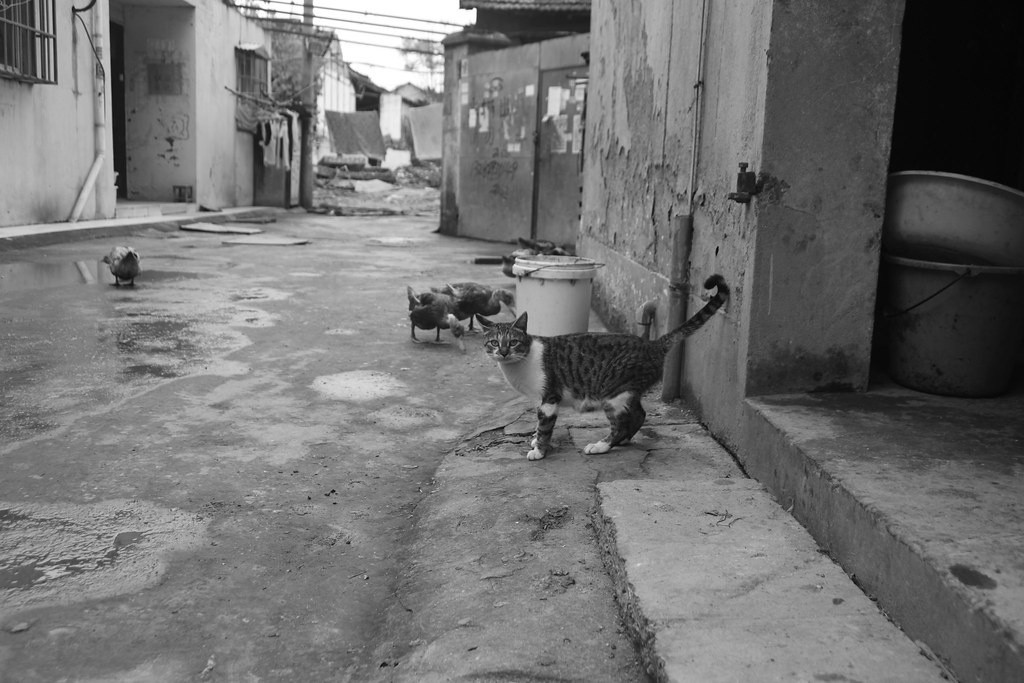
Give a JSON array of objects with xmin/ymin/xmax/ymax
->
[{"xmin": 882, "ymin": 170, "xmax": 1023, "ymax": 264}]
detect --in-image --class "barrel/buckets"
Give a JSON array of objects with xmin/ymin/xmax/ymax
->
[
  {"xmin": 888, "ymin": 251, "xmax": 1024, "ymax": 398},
  {"xmin": 511, "ymin": 255, "xmax": 597, "ymax": 337}
]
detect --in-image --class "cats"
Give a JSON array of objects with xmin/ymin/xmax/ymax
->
[{"xmin": 474, "ymin": 275, "xmax": 731, "ymax": 461}]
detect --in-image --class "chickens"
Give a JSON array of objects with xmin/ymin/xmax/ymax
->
[{"xmin": 102, "ymin": 245, "xmax": 142, "ymax": 287}]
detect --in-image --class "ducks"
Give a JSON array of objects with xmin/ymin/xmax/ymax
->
[
  {"xmin": 432, "ymin": 281, "xmax": 515, "ymax": 334},
  {"xmin": 407, "ymin": 285, "xmax": 466, "ymax": 352}
]
